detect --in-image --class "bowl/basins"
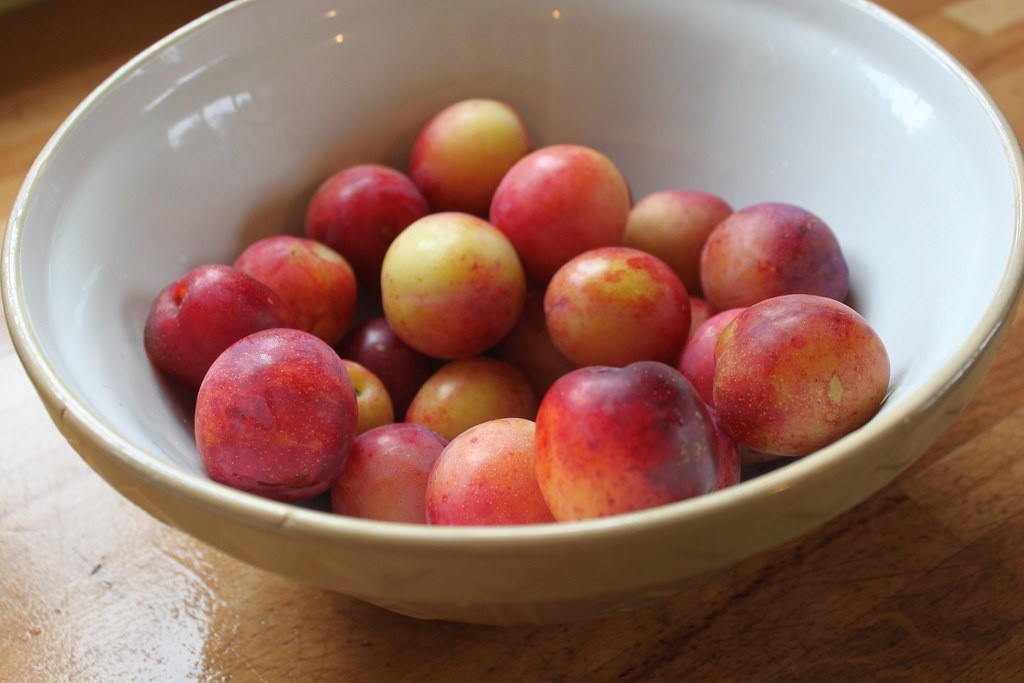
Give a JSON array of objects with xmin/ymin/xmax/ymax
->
[{"xmin": 0, "ymin": 0, "xmax": 1024, "ymax": 619}]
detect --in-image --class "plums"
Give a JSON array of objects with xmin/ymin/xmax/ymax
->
[{"xmin": 144, "ymin": 99, "xmax": 889, "ymax": 527}]
